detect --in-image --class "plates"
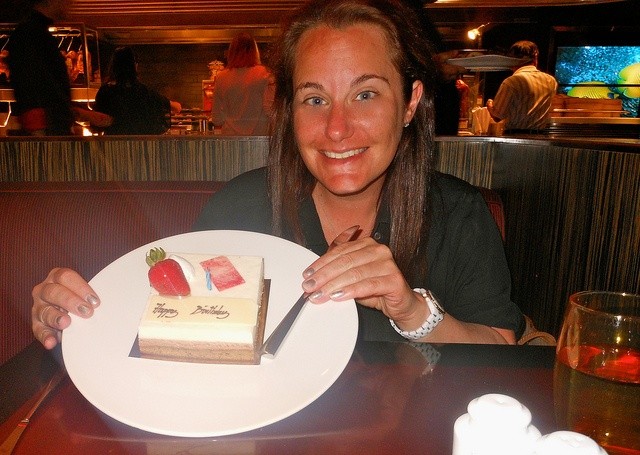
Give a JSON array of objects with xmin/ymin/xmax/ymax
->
[
  {"xmin": 61, "ymin": 229, "xmax": 358, "ymax": 438},
  {"xmin": 445, "ymin": 53, "xmax": 532, "ymax": 73}
]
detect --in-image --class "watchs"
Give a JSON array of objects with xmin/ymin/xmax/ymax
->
[{"xmin": 389, "ymin": 288, "xmax": 445, "ymax": 340}]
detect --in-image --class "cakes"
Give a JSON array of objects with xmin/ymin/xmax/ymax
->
[{"xmin": 137, "ymin": 247, "xmax": 264, "ymax": 365}]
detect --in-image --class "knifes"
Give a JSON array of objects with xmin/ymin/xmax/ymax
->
[
  {"xmin": 257, "ymin": 225, "xmax": 360, "ymax": 359},
  {"xmin": 0, "ymin": 367, "xmax": 67, "ymax": 455}
]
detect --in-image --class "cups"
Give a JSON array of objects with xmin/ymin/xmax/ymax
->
[{"xmin": 553, "ymin": 290, "xmax": 640, "ymax": 455}]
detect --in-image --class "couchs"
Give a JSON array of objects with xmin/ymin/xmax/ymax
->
[{"xmin": 0, "ymin": 180, "xmax": 505, "ymax": 359}]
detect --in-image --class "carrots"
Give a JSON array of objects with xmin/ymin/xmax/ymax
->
[{"xmin": 145, "ymin": 245, "xmax": 192, "ymax": 299}]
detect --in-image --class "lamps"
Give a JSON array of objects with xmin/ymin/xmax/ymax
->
[{"xmin": 467, "ymin": 21, "xmax": 491, "ymax": 41}]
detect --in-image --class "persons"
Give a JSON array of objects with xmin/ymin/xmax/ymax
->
[
  {"xmin": 31, "ymin": 0, "xmax": 521, "ymax": 349},
  {"xmin": 212, "ymin": 30, "xmax": 276, "ymax": 136},
  {"xmin": 89, "ymin": 47, "xmax": 172, "ymax": 135},
  {"xmin": 487, "ymin": 41, "xmax": 558, "ymax": 135}
]
